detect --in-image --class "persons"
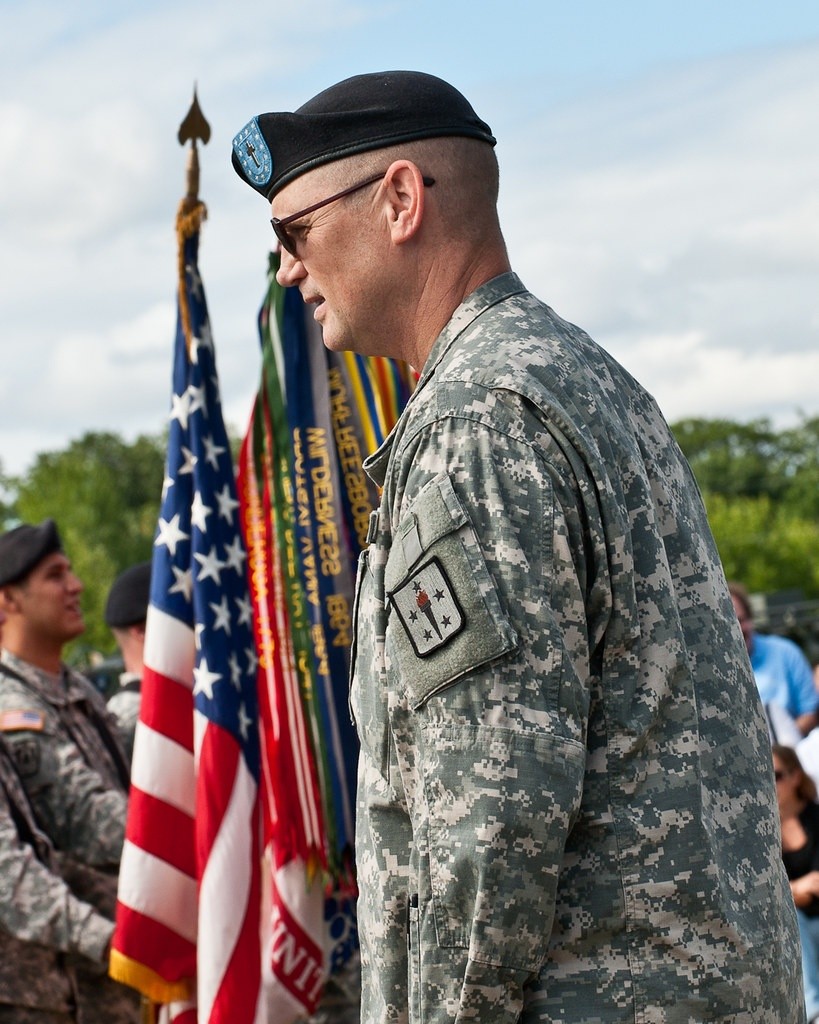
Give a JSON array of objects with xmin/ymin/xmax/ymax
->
[
  {"xmin": 0, "ymin": 743, "xmax": 119, "ymax": 1024},
  {"xmin": 231, "ymin": 69, "xmax": 806, "ymax": 1024},
  {"xmin": 106, "ymin": 568, "xmax": 153, "ymax": 778},
  {"xmin": 770, "ymin": 745, "xmax": 819, "ymax": 1024},
  {"xmin": 725, "ymin": 583, "xmax": 818, "ymax": 738},
  {"xmin": 0, "ymin": 516, "xmax": 150, "ymax": 1023}
]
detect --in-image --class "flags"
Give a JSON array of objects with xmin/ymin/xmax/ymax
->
[{"xmin": 109, "ymin": 201, "xmax": 423, "ymax": 1024}]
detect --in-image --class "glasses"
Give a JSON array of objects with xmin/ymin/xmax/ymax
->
[
  {"xmin": 270, "ymin": 171, "xmax": 437, "ymax": 255},
  {"xmin": 773, "ymin": 769, "xmax": 793, "ymax": 781}
]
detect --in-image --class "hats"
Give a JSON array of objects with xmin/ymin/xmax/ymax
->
[
  {"xmin": 0, "ymin": 521, "xmax": 59, "ymax": 582},
  {"xmin": 106, "ymin": 560, "xmax": 153, "ymax": 627},
  {"xmin": 229, "ymin": 71, "xmax": 495, "ymax": 204}
]
